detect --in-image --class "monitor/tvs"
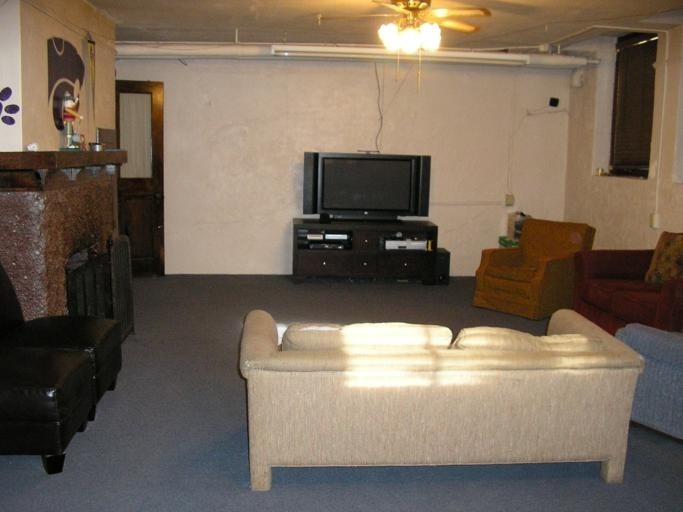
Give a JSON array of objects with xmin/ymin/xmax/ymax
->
[{"xmin": 316, "ymin": 152, "xmax": 421, "ymax": 221}]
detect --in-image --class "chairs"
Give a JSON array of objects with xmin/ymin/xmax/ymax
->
[
  {"xmin": 615, "ymin": 323, "xmax": 683, "ymax": 439},
  {"xmin": 472, "ymin": 219, "xmax": 596, "ymax": 321},
  {"xmin": 0, "ymin": 262, "xmax": 122, "ymax": 474},
  {"xmin": 572, "ymin": 231, "xmax": 683, "ymax": 336}
]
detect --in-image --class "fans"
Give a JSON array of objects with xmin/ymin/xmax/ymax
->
[{"xmin": 314, "ymin": 0, "xmax": 492, "ymax": 33}]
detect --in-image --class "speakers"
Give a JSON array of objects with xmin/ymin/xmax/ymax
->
[
  {"xmin": 302, "ymin": 151, "xmax": 318, "ymax": 215},
  {"xmin": 417, "ymin": 155, "xmax": 432, "ymax": 217}
]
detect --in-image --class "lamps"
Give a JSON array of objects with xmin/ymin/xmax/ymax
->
[
  {"xmin": 378, "ymin": 16, "xmax": 441, "ymax": 53},
  {"xmin": 271, "ymin": 44, "xmax": 600, "ymax": 69}
]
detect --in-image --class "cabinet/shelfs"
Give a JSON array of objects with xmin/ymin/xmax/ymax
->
[{"xmin": 293, "ymin": 219, "xmax": 437, "ymax": 285}]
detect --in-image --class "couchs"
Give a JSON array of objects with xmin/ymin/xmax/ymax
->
[{"xmin": 239, "ymin": 308, "xmax": 645, "ymax": 491}]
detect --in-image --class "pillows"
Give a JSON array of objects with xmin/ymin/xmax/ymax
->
[
  {"xmin": 282, "ymin": 322, "xmax": 453, "ymax": 354},
  {"xmin": 644, "ymin": 231, "xmax": 683, "ymax": 285},
  {"xmin": 449, "ymin": 325, "xmax": 594, "ymax": 355}
]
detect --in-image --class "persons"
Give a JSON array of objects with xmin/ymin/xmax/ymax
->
[{"xmin": 59, "ymin": 90, "xmax": 85, "ymax": 151}]
